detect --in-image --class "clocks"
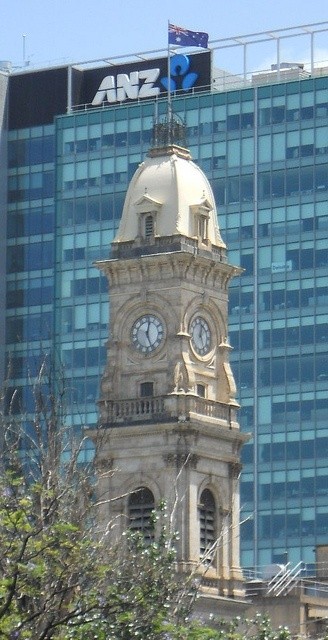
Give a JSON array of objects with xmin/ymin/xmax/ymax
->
[
  {"xmin": 125, "ymin": 310, "xmax": 166, "ymax": 359},
  {"xmin": 187, "ymin": 308, "xmax": 219, "ymax": 361}
]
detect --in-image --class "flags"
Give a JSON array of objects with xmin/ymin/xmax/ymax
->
[{"xmin": 168, "ymin": 23, "xmax": 209, "ymax": 50}]
